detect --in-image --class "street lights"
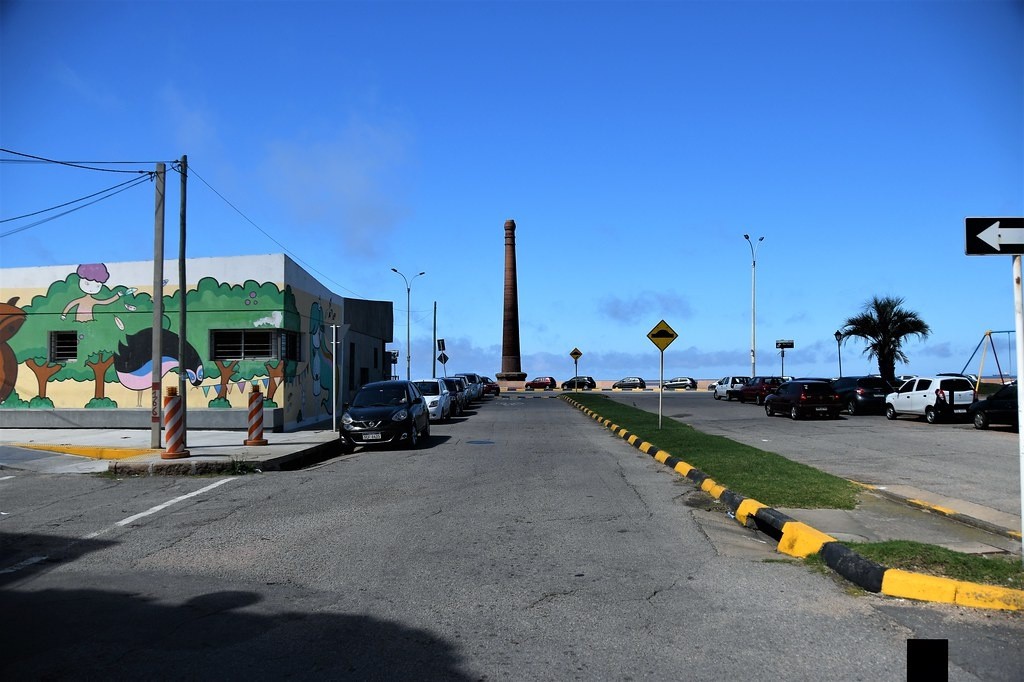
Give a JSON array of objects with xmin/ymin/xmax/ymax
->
[
  {"xmin": 743, "ymin": 234, "xmax": 764, "ymax": 378},
  {"xmin": 834, "ymin": 329, "xmax": 843, "ymax": 378},
  {"xmin": 390, "ymin": 267, "xmax": 426, "ymax": 382}
]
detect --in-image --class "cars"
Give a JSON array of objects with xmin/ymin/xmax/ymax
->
[
  {"xmin": 764, "ymin": 376, "xmax": 896, "ymax": 420},
  {"xmin": 885, "ymin": 373, "xmax": 978, "ymax": 424},
  {"xmin": 561, "ymin": 376, "xmax": 596, "ymax": 391},
  {"xmin": 525, "ymin": 377, "xmax": 557, "ymax": 389},
  {"xmin": 612, "ymin": 377, "xmax": 646, "ymax": 389},
  {"xmin": 339, "ymin": 380, "xmax": 431, "ymax": 454},
  {"xmin": 966, "ymin": 380, "xmax": 1019, "ymax": 432},
  {"xmin": 659, "ymin": 377, "xmax": 698, "ymax": 391},
  {"xmin": 708, "ymin": 376, "xmax": 786, "ymax": 406},
  {"xmin": 404, "ymin": 372, "xmax": 501, "ymax": 423}
]
